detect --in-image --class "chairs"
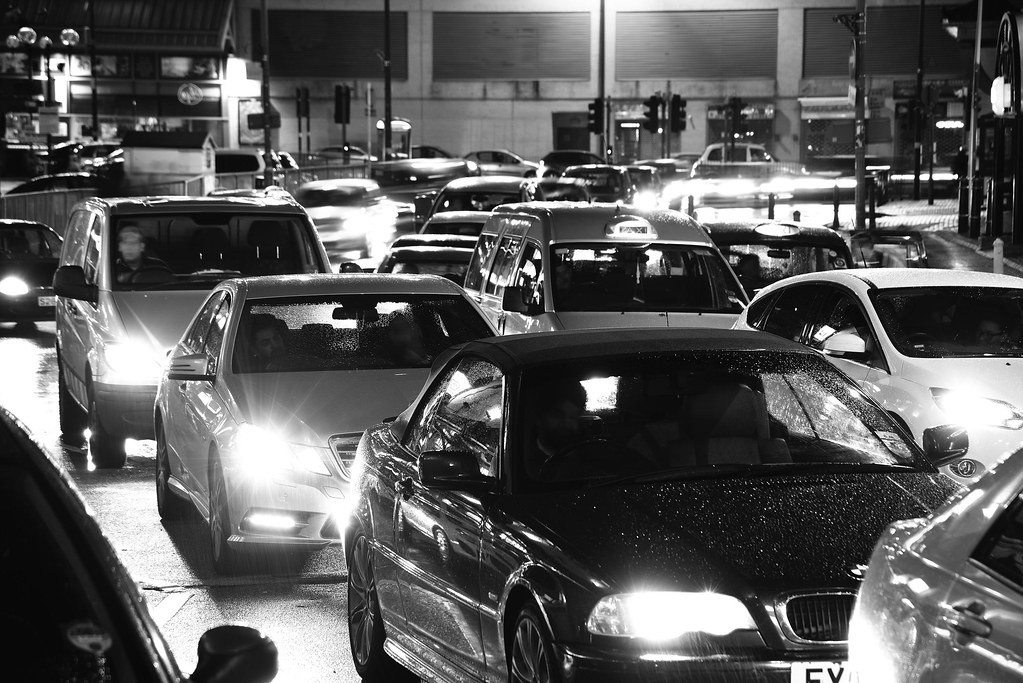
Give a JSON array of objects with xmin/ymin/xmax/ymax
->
[
  {"xmin": 666, "ymin": 386, "xmax": 795, "ymax": 473},
  {"xmin": 640, "ymin": 275, "xmax": 704, "ymax": 308},
  {"xmin": 116, "ymin": 220, "xmax": 285, "ymax": 270}
]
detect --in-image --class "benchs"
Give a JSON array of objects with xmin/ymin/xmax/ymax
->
[{"xmin": 281, "ymin": 319, "xmax": 362, "ymax": 360}]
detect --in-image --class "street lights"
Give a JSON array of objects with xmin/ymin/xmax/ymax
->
[{"xmin": 9, "ymin": 23, "xmax": 78, "ymax": 132}]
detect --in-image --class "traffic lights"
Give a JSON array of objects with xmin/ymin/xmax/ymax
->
[
  {"xmin": 738, "ymin": 101, "xmax": 748, "ymax": 135},
  {"xmin": 333, "ymin": 84, "xmax": 346, "ymax": 123},
  {"xmin": 673, "ymin": 96, "xmax": 688, "ymax": 133},
  {"xmin": 586, "ymin": 102, "xmax": 599, "ymax": 133},
  {"xmin": 642, "ymin": 99, "xmax": 655, "ymax": 132},
  {"xmin": 296, "ymin": 83, "xmax": 308, "ymax": 120}
]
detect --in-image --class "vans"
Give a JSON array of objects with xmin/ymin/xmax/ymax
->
[
  {"xmin": 461, "ymin": 200, "xmax": 757, "ymax": 336},
  {"xmin": 50, "ymin": 186, "xmax": 364, "ymax": 474}
]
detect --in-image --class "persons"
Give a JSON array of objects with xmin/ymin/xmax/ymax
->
[
  {"xmin": 251, "ymin": 320, "xmax": 286, "ymax": 362},
  {"xmin": 114, "ymin": 226, "xmax": 171, "ymax": 283},
  {"xmin": 529, "ymin": 370, "xmax": 585, "ymax": 478}
]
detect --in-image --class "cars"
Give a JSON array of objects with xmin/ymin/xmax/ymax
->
[
  {"xmin": 0, "ymin": 408, "xmax": 280, "ymax": 682},
  {"xmin": 1, "ymin": 126, "xmax": 857, "ymax": 341},
  {"xmin": 152, "ymin": 273, "xmax": 543, "ymax": 585},
  {"xmin": 848, "ymin": 452, "xmax": 1023, "ymax": 683},
  {"xmin": 730, "ymin": 265, "xmax": 1023, "ymax": 484},
  {"xmin": 335, "ymin": 328, "xmax": 969, "ymax": 682}
]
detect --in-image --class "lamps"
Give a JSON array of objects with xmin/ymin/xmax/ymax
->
[{"xmin": 990, "ymin": 75, "xmax": 1011, "ymax": 117}]
